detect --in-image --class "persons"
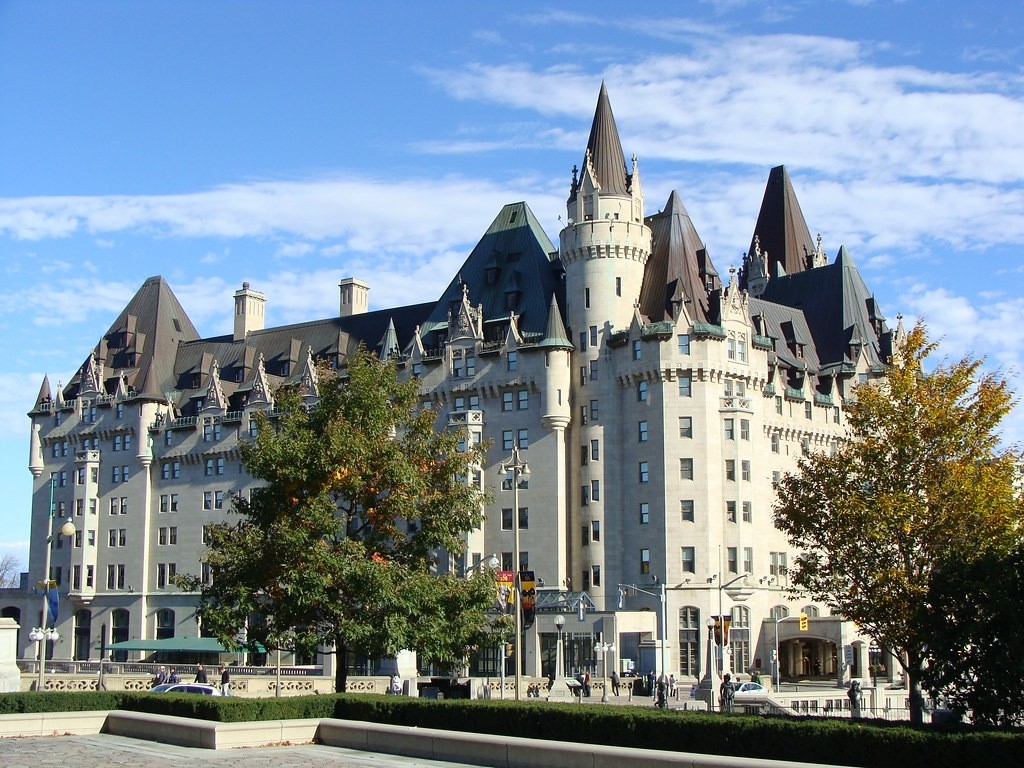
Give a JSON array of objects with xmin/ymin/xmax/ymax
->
[
  {"xmin": 848, "ymin": 680, "xmax": 863, "ymax": 705},
  {"xmin": 527, "ymin": 683, "xmax": 541, "ymax": 697},
  {"xmin": 577, "ymin": 671, "xmax": 591, "ymax": 697},
  {"xmin": 647, "ymin": 670, "xmax": 654, "ymax": 697},
  {"xmin": 390, "ymin": 673, "xmax": 401, "ymax": 696},
  {"xmin": 497, "ymin": 586, "xmax": 514, "ymax": 614},
  {"xmin": 670, "ymin": 675, "xmax": 677, "ymax": 697},
  {"xmin": 220, "ymin": 666, "xmax": 230, "ymax": 697},
  {"xmin": 151, "ymin": 666, "xmax": 167, "ymax": 690},
  {"xmin": 543, "ymin": 682, "xmax": 549, "ymax": 690},
  {"xmin": 751, "ymin": 670, "xmax": 762, "ymax": 688},
  {"xmin": 610, "ymin": 671, "xmax": 620, "ymax": 696},
  {"xmin": 194, "ymin": 662, "xmax": 207, "ymax": 685},
  {"xmin": 457, "ymin": 671, "xmax": 463, "ymax": 678},
  {"xmin": 720, "ymin": 674, "xmax": 735, "ymax": 714},
  {"xmin": 417, "ymin": 671, "xmax": 423, "ymax": 677},
  {"xmin": 169, "ymin": 667, "xmax": 178, "ymax": 684}
]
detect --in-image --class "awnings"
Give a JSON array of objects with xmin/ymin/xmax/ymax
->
[{"xmin": 95, "ymin": 637, "xmax": 266, "ymax": 653}]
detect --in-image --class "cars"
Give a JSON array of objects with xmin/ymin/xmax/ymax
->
[
  {"xmin": 146, "ymin": 684, "xmax": 220, "ymax": 697},
  {"xmin": 732, "ymin": 682, "xmax": 770, "ymax": 699}
]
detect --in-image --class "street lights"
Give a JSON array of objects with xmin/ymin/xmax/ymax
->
[
  {"xmin": 498, "ymin": 445, "xmax": 533, "ymax": 701},
  {"xmin": 617, "ymin": 583, "xmax": 666, "ymax": 682},
  {"xmin": 869, "ymin": 640, "xmax": 879, "ymax": 688},
  {"xmin": 548, "ymin": 616, "xmax": 576, "ymax": 703},
  {"xmin": 694, "ymin": 617, "xmax": 724, "ymax": 711},
  {"xmin": 500, "ymin": 597, "xmax": 586, "ymax": 700},
  {"xmin": 462, "ymin": 553, "xmax": 499, "ymax": 679},
  {"xmin": 718, "ymin": 544, "xmax": 752, "ymax": 676},
  {"xmin": 39, "ymin": 477, "xmax": 77, "ymax": 692}
]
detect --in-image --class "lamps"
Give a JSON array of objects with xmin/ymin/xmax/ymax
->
[
  {"xmin": 128, "ymin": 586, "xmax": 135, "ymax": 592},
  {"xmin": 759, "ymin": 575, "xmax": 767, "ymax": 584},
  {"xmin": 680, "ymin": 578, "xmax": 691, "ymax": 585},
  {"xmin": 538, "ymin": 577, "xmax": 546, "ymax": 585},
  {"xmin": 709, "ymin": 574, "xmax": 717, "ymax": 584},
  {"xmin": 651, "ymin": 575, "xmax": 658, "ymax": 583},
  {"xmin": 566, "ymin": 577, "xmax": 571, "ymax": 585},
  {"xmin": 768, "ymin": 577, "xmax": 775, "ymax": 586}
]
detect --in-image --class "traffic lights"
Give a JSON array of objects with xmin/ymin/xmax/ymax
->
[{"xmin": 800, "ymin": 613, "xmax": 808, "ymax": 630}]
